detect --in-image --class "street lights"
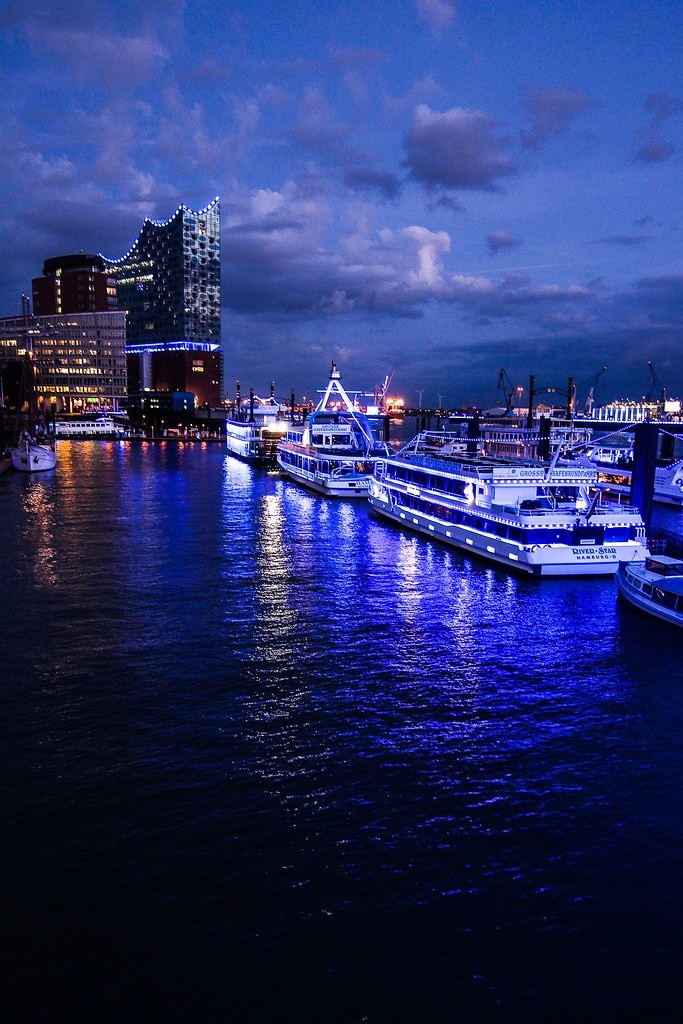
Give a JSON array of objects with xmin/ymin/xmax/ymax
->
[{"xmin": 141, "ymin": 415, "xmax": 220, "ymax": 440}]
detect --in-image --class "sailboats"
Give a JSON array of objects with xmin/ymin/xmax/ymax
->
[{"xmin": 9, "ymin": 352, "xmax": 57, "ymax": 471}]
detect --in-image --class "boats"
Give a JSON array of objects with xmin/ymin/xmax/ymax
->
[
  {"xmin": 476, "ymin": 425, "xmax": 682, "ymax": 505},
  {"xmin": 49, "ymin": 417, "xmax": 132, "ymax": 439},
  {"xmin": 366, "ymin": 405, "xmax": 653, "ymax": 582},
  {"xmin": 275, "ymin": 361, "xmax": 396, "ymax": 500},
  {"xmin": 224, "ymin": 379, "xmax": 315, "ymax": 459},
  {"xmin": 614, "ymin": 555, "xmax": 683, "ymax": 628}
]
